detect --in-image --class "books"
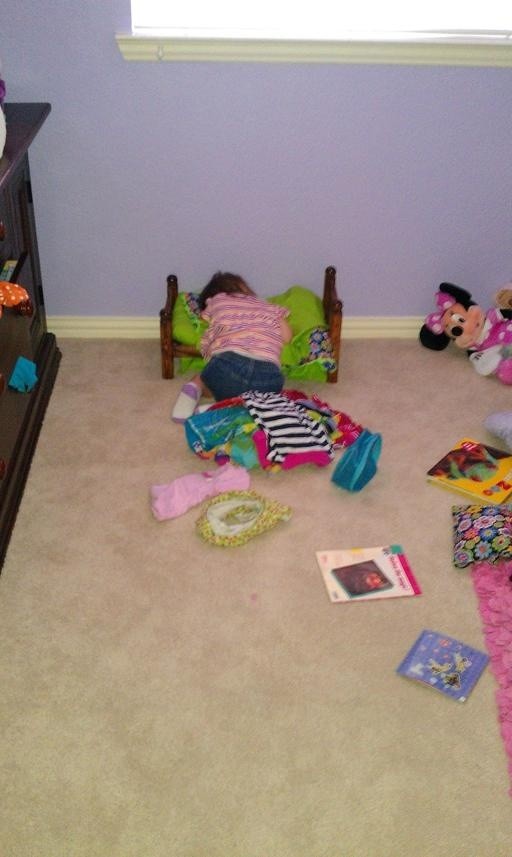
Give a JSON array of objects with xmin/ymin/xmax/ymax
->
[
  {"xmin": 316, "ymin": 542, "xmax": 422, "ymax": 602},
  {"xmin": 426, "ymin": 436, "xmax": 511, "ymax": 505},
  {"xmin": 398, "ymin": 630, "xmax": 492, "ymax": 704}
]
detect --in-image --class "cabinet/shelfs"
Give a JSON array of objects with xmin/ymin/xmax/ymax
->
[{"xmin": 0, "ymin": 100, "xmax": 64, "ymax": 575}]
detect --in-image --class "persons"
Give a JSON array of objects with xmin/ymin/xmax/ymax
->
[{"xmin": 170, "ymin": 272, "xmax": 293, "ymax": 423}]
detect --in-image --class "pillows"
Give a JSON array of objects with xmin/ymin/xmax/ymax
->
[{"xmin": 448, "ymin": 502, "xmax": 512, "ymax": 571}]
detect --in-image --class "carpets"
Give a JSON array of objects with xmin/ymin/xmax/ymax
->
[{"xmin": 461, "ymin": 555, "xmax": 512, "ymax": 798}]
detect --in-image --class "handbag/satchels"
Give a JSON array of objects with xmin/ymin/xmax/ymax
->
[{"xmin": 331, "ymin": 428, "xmax": 382, "ymax": 494}]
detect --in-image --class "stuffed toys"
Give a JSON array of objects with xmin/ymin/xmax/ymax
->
[{"xmin": 419, "ymin": 280, "xmax": 512, "ymax": 386}]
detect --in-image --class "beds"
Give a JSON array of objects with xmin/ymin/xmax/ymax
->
[{"xmin": 157, "ymin": 264, "xmax": 343, "ymax": 385}]
